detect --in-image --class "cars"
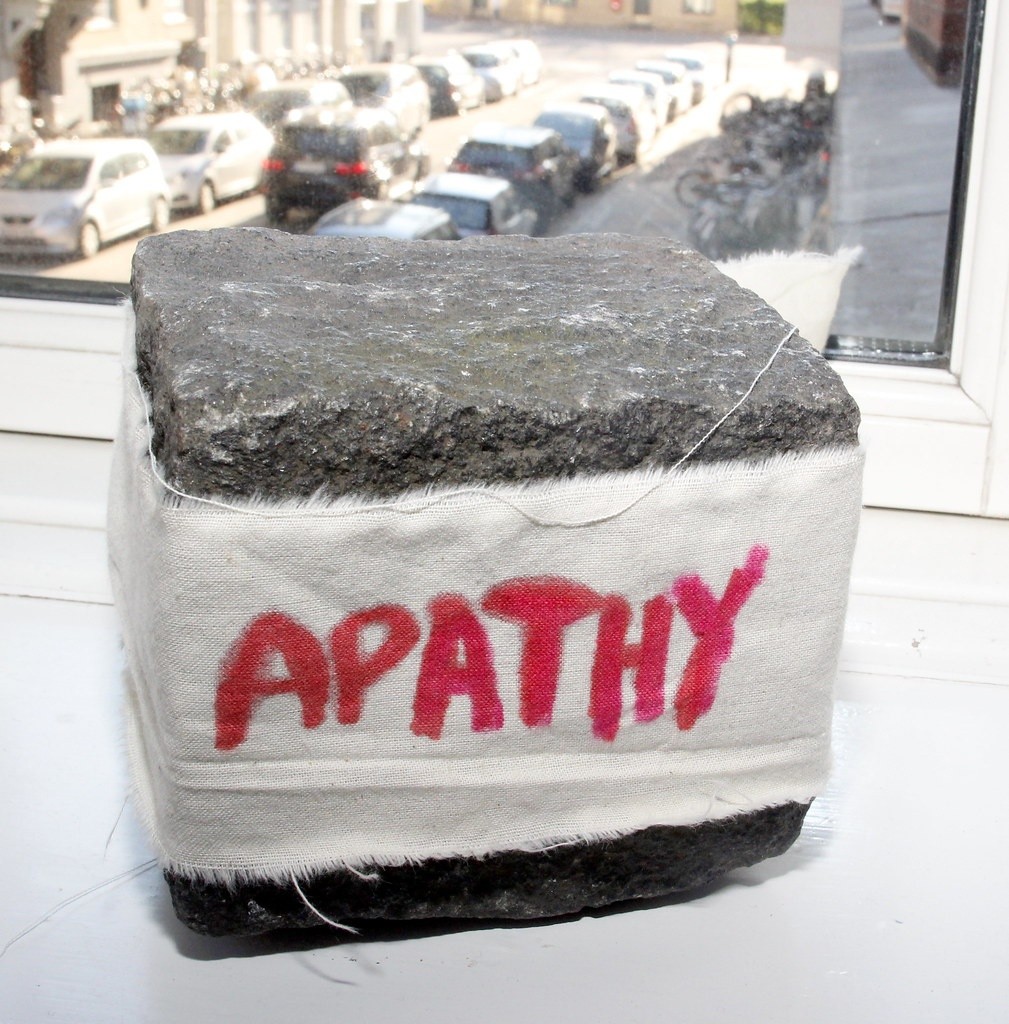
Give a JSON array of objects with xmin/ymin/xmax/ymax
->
[
  {"xmin": 146, "ymin": 110, "xmax": 278, "ymax": 215},
  {"xmin": 261, "ymin": 100, "xmax": 434, "ymax": 226},
  {"xmin": 532, "ymin": 98, "xmax": 620, "ymax": 195},
  {"xmin": 302, "ymin": 195, "xmax": 464, "ymax": 242},
  {"xmin": 399, "ymin": 170, "xmax": 540, "ymax": 238},
  {"xmin": 244, "ymin": 34, "xmax": 842, "ymax": 262},
  {"xmin": 448, "ymin": 120, "xmax": 584, "ymax": 229},
  {"xmin": 0, "ymin": 139, "xmax": 176, "ymax": 262}
]
